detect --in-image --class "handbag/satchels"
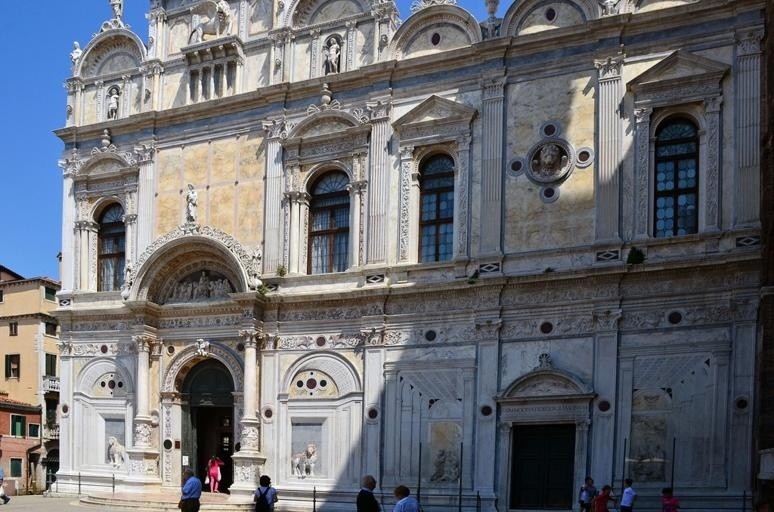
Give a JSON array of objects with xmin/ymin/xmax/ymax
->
[{"xmin": 204, "ymin": 476, "xmax": 209, "ymax": 484}]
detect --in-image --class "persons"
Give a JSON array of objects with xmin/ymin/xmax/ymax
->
[
  {"xmin": 356, "ymin": 475, "xmax": 381, "ymax": 512},
  {"xmin": 323, "ymin": 37, "xmax": 340, "ymax": 76},
  {"xmin": 578, "ymin": 477, "xmax": 637, "ymax": 512},
  {"xmin": 661, "ymin": 487, "xmax": 680, "ymax": 512},
  {"xmin": 0, "ymin": 467, "xmax": 10, "ymax": 505},
  {"xmin": 392, "ymin": 485, "xmax": 419, "ymax": 512},
  {"xmin": 254, "ymin": 475, "xmax": 278, "ymax": 512},
  {"xmin": 208, "ymin": 454, "xmax": 225, "ymax": 493},
  {"xmin": 178, "ymin": 468, "xmax": 202, "ymax": 512},
  {"xmin": 106, "ymin": 88, "xmax": 122, "ymax": 120},
  {"xmin": 172, "ymin": 271, "xmax": 232, "ymax": 299},
  {"xmin": 186, "ymin": 183, "xmax": 197, "ymax": 224},
  {"xmin": 70, "ymin": 41, "xmax": 83, "ymax": 66},
  {"xmin": 109, "ymin": 0, "xmax": 124, "ymax": 20}
]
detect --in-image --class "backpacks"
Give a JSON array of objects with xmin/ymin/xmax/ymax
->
[{"xmin": 255, "ymin": 487, "xmax": 270, "ymax": 512}]
